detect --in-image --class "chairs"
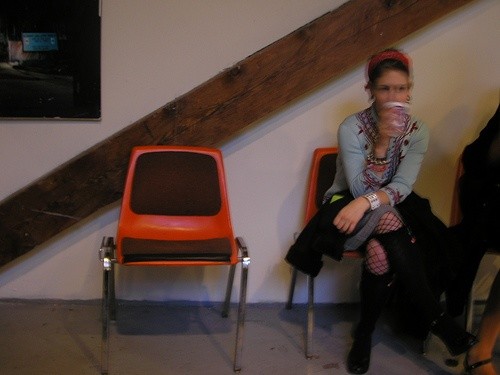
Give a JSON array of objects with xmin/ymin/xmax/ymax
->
[
  {"xmin": 284, "ymin": 148, "xmax": 429, "ymax": 361},
  {"xmin": 99, "ymin": 146, "xmax": 249, "ymax": 375}
]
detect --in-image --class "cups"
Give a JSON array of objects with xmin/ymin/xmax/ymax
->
[{"xmin": 384, "ymin": 102, "xmax": 409, "ymax": 135}]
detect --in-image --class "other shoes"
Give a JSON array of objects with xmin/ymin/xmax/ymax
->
[
  {"xmin": 345, "ymin": 305, "xmax": 379, "ymax": 375},
  {"xmin": 430, "ymin": 319, "xmax": 478, "ymax": 356}
]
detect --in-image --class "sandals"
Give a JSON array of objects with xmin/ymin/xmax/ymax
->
[{"xmin": 463, "ymin": 346, "xmax": 498, "ymax": 375}]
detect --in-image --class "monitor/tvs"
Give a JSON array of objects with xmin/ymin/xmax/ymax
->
[
  {"xmin": 21, "ymin": 33, "xmax": 59, "ymax": 53},
  {"xmin": 0, "ymin": 0, "xmax": 101, "ymax": 121}
]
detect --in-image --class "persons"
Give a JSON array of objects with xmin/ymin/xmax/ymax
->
[
  {"xmin": 323, "ymin": 49, "xmax": 480, "ymax": 375},
  {"xmin": 458, "ymin": 102, "xmax": 500, "ymax": 375}
]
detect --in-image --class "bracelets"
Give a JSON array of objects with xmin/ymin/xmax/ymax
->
[
  {"xmin": 366, "ymin": 155, "xmax": 388, "ymax": 172},
  {"xmin": 361, "ymin": 191, "xmax": 383, "ymax": 213}
]
